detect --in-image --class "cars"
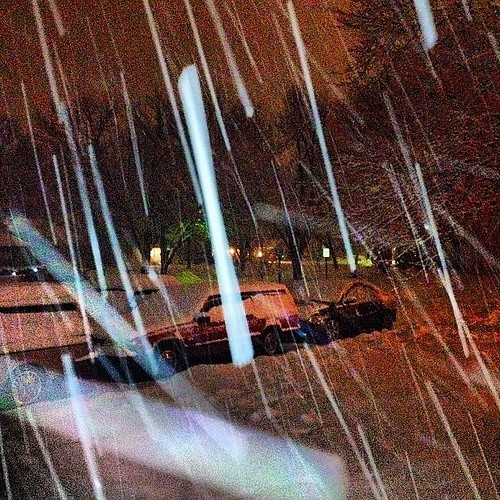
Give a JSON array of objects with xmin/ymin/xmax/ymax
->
[{"xmin": 290, "ymin": 278, "xmax": 397, "ymax": 345}]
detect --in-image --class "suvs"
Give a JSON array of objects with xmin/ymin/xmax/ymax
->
[{"xmin": 94, "ymin": 282, "xmax": 299, "ymax": 380}]
